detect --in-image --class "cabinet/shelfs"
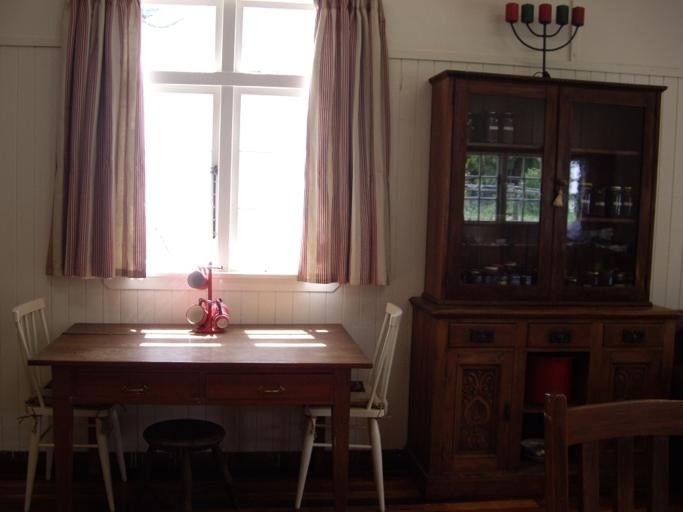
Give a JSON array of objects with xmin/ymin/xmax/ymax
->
[
  {"xmin": 422, "ymin": 70, "xmax": 669, "ymax": 308},
  {"xmin": 403, "ymin": 297, "xmax": 683, "ymax": 502}
]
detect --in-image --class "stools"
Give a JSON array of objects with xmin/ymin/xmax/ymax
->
[{"xmin": 134, "ymin": 418, "xmax": 243, "ymax": 511}]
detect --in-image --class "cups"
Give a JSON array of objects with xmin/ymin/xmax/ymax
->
[
  {"xmin": 187, "ymin": 266, "xmax": 209, "ymax": 291},
  {"xmin": 184, "ymin": 296, "xmax": 208, "ymax": 327},
  {"xmin": 210, "ymin": 299, "xmax": 232, "ymax": 329}
]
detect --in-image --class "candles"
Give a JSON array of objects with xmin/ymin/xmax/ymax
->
[{"xmin": 506, "ymin": 3, "xmax": 585, "ymax": 25}]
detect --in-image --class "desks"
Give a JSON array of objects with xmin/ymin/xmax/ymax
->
[{"xmin": 26, "ymin": 324, "xmax": 373, "ymax": 511}]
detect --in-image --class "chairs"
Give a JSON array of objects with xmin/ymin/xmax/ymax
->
[
  {"xmin": 543, "ymin": 393, "xmax": 683, "ymax": 511},
  {"xmin": 294, "ymin": 302, "xmax": 403, "ymax": 511},
  {"xmin": 11, "ymin": 296, "xmax": 127, "ymax": 511}
]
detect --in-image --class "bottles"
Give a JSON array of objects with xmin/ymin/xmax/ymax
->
[
  {"xmin": 567, "ymin": 267, "xmax": 632, "ymax": 288},
  {"xmin": 575, "ymin": 181, "xmax": 635, "ymax": 219},
  {"xmin": 466, "ymin": 262, "xmax": 534, "ymax": 285}
]
